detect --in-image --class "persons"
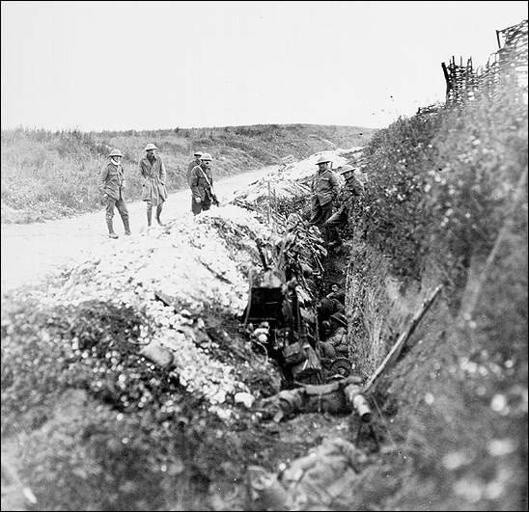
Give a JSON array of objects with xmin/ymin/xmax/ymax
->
[
  {"xmin": 134, "ymin": 143, "xmax": 168, "ymax": 227},
  {"xmin": 322, "ymin": 165, "xmax": 365, "ymax": 248},
  {"xmin": 188, "ymin": 153, "xmax": 213, "ymax": 216},
  {"xmin": 315, "ymin": 280, "xmax": 345, "ymax": 319},
  {"xmin": 187, "ymin": 151, "xmax": 203, "ymax": 188},
  {"xmin": 96, "ymin": 149, "xmax": 133, "ymax": 239},
  {"xmin": 311, "ymin": 156, "xmax": 347, "ymax": 253}
]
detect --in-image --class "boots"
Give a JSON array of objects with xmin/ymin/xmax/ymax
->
[
  {"xmin": 146, "ymin": 208, "xmax": 162, "ymax": 225},
  {"xmin": 107, "ymin": 222, "xmax": 118, "ymax": 238},
  {"xmin": 328, "ymin": 229, "xmax": 342, "ymax": 247},
  {"xmin": 123, "ymin": 219, "xmax": 132, "ymax": 236}
]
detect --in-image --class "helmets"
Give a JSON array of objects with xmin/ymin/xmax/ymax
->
[
  {"xmin": 341, "ymin": 164, "xmax": 355, "ymax": 175},
  {"xmin": 194, "ymin": 151, "xmax": 204, "ymax": 156},
  {"xmin": 201, "ymin": 153, "xmax": 213, "ymax": 161},
  {"xmin": 330, "ymin": 313, "xmax": 347, "ymax": 325},
  {"xmin": 315, "ymin": 158, "xmax": 330, "ymax": 165},
  {"xmin": 144, "ymin": 144, "xmax": 157, "ymax": 151},
  {"xmin": 109, "ymin": 150, "xmax": 124, "ymax": 157}
]
